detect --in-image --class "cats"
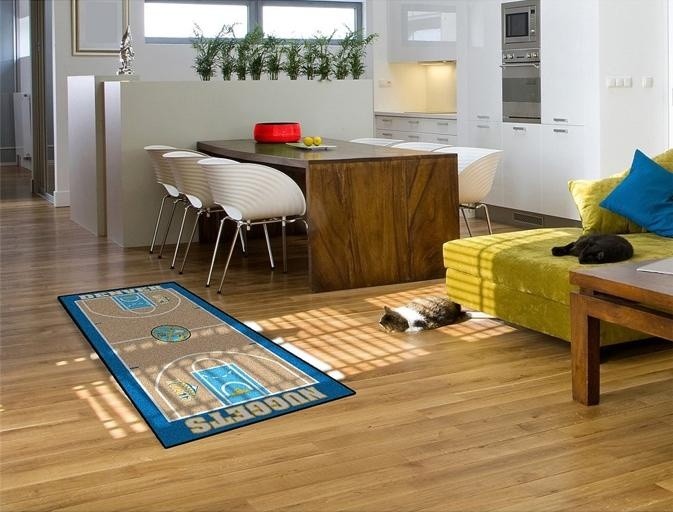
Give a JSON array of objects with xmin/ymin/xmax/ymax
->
[
  {"xmin": 552, "ymin": 227, "xmax": 634, "ymax": 265},
  {"xmin": 379, "ymin": 295, "xmax": 462, "ymax": 336}
]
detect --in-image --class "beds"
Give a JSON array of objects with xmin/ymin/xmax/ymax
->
[{"xmin": 443, "ymin": 227, "xmax": 673, "ymax": 361}]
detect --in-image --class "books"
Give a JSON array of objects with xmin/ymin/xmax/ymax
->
[{"xmin": 635, "ymin": 259, "xmax": 673, "ymax": 275}]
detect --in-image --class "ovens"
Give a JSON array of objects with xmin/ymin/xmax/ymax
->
[{"xmin": 501, "ymin": 50, "xmax": 541, "ymax": 124}]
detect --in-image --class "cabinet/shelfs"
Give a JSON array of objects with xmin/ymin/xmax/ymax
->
[
  {"xmin": 541, "ymin": 125, "xmax": 670, "ymax": 223},
  {"xmin": 464, "ymin": 1, "xmax": 502, "ymax": 121},
  {"xmin": 458, "ymin": 121, "xmax": 503, "ymax": 208},
  {"xmin": 399, "ymin": 116, "xmax": 423, "ymax": 142},
  {"xmin": 502, "ymin": 122, "xmax": 541, "ymax": 214},
  {"xmin": 374, "ymin": 114, "xmax": 400, "ymax": 138},
  {"xmin": 541, "ymin": 0, "xmax": 668, "ymax": 125},
  {"xmin": 57, "ymin": 281, "xmax": 357, "ymax": 448},
  {"xmin": 422, "ymin": 118, "xmax": 456, "ymax": 147}
]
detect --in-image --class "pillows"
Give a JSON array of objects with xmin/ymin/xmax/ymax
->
[
  {"xmin": 620, "ymin": 148, "xmax": 673, "ymax": 176},
  {"xmin": 567, "ymin": 177, "xmax": 647, "ymax": 236},
  {"xmin": 599, "ymin": 149, "xmax": 673, "ymax": 238}
]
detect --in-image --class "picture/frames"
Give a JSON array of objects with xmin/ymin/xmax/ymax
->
[{"xmin": 70, "ymin": 0, "xmax": 130, "ymax": 58}]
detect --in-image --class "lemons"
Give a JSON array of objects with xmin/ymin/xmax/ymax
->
[
  {"xmin": 303, "ymin": 136, "xmax": 322, "ymax": 146},
  {"xmin": 305, "ymin": 152, "xmax": 320, "ymax": 160}
]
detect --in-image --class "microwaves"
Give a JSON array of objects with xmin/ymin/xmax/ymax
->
[{"xmin": 500, "ymin": 1, "xmax": 539, "ymax": 49}]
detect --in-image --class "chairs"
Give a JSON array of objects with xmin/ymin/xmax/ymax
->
[
  {"xmin": 436, "ymin": 147, "xmax": 504, "ymax": 236},
  {"xmin": 349, "ymin": 138, "xmax": 405, "ymax": 147},
  {"xmin": 393, "ymin": 142, "xmax": 453, "ymax": 151},
  {"xmin": 144, "ymin": 143, "xmax": 212, "ymax": 259},
  {"xmin": 198, "ymin": 157, "xmax": 308, "ymax": 293},
  {"xmin": 162, "ymin": 152, "xmax": 247, "ymax": 274}
]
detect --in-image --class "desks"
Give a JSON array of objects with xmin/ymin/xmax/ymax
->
[{"xmin": 197, "ymin": 139, "xmax": 461, "ymax": 294}]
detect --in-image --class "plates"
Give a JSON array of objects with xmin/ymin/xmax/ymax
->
[{"xmin": 285, "ymin": 141, "xmax": 336, "ymax": 150}]
